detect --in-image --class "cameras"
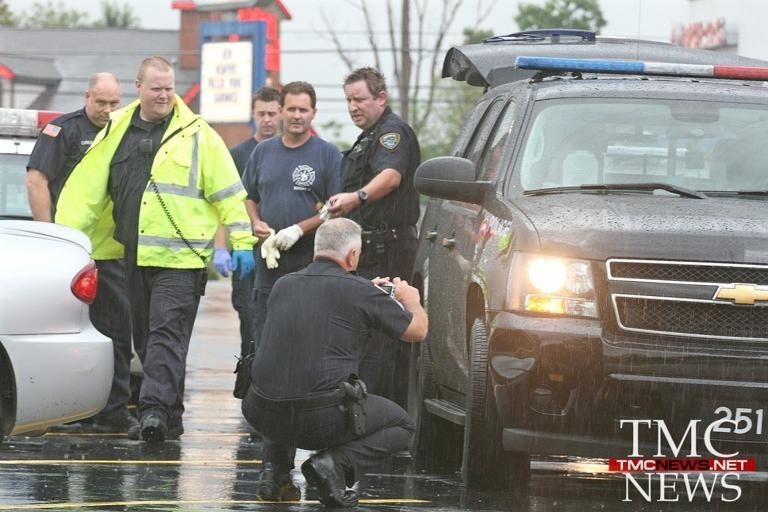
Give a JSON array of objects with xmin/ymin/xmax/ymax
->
[{"xmin": 378, "ymin": 285, "xmax": 395, "ymax": 298}]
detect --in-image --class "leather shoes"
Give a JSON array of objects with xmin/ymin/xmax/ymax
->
[
  {"xmin": 82, "ymin": 412, "xmax": 135, "ymax": 433},
  {"xmin": 138, "ymin": 411, "xmax": 168, "ymax": 442},
  {"xmin": 256, "ymin": 468, "xmax": 302, "ymax": 501},
  {"xmin": 127, "ymin": 416, "xmax": 184, "ymax": 441},
  {"xmin": 302, "ymin": 450, "xmax": 359, "ymax": 508}
]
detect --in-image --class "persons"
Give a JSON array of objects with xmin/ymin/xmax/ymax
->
[
  {"xmin": 26, "ymin": 70, "xmax": 142, "ymax": 435},
  {"xmin": 321, "ymin": 66, "xmax": 426, "ymax": 412},
  {"xmin": 240, "ymin": 216, "xmax": 432, "ymax": 508},
  {"xmin": 54, "ymin": 57, "xmax": 258, "ymax": 441},
  {"xmin": 210, "ymin": 84, "xmax": 285, "ymax": 351},
  {"xmin": 239, "ymin": 80, "xmax": 347, "ymax": 350}
]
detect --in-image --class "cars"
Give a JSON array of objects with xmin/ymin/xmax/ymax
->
[
  {"xmin": 0, "ymin": 213, "xmax": 115, "ymax": 453},
  {"xmin": 0, "ymin": 105, "xmax": 72, "ymax": 217}
]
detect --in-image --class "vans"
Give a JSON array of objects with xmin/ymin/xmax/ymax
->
[{"xmin": 409, "ymin": 30, "xmax": 767, "ymax": 510}]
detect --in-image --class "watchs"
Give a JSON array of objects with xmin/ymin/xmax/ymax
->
[{"xmin": 356, "ymin": 188, "xmax": 368, "ymax": 210}]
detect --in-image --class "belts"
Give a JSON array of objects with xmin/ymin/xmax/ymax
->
[
  {"xmin": 249, "ymin": 385, "xmax": 345, "ymax": 414},
  {"xmin": 363, "ymin": 226, "xmax": 418, "ymax": 245}
]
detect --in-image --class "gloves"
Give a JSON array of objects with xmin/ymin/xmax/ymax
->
[
  {"xmin": 230, "ymin": 250, "xmax": 255, "ymax": 280},
  {"xmin": 261, "ymin": 229, "xmax": 281, "ymax": 270},
  {"xmin": 271, "ymin": 224, "xmax": 304, "ymax": 252},
  {"xmin": 319, "ymin": 200, "xmax": 334, "ymax": 221},
  {"xmin": 211, "ymin": 246, "xmax": 232, "ymax": 278}
]
